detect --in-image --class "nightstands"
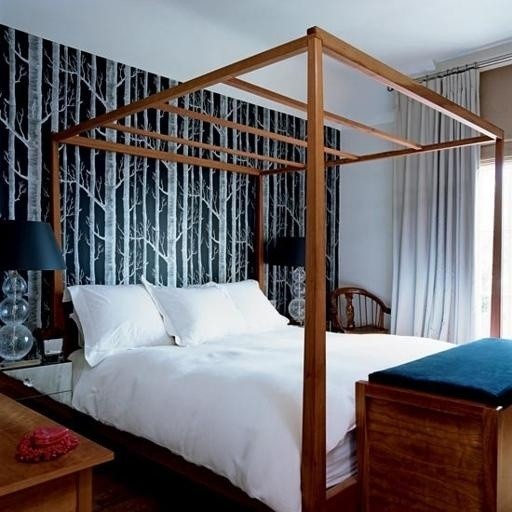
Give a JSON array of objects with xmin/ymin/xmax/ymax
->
[{"xmin": 1, "ymin": 353, "xmax": 72, "ymax": 405}]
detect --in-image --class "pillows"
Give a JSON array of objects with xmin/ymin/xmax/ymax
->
[
  {"xmin": 141, "ymin": 275, "xmax": 290, "ymax": 346},
  {"xmin": 62, "ymin": 283, "xmax": 175, "ymax": 366}
]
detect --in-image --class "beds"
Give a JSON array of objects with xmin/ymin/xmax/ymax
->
[{"xmin": 48, "ymin": 27, "xmax": 503, "ymax": 512}]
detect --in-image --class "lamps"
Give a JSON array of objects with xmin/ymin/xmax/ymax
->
[
  {"xmin": 0, "ymin": 219, "xmax": 66, "ymax": 370},
  {"xmin": 269, "ymin": 236, "xmax": 306, "ymax": 325}
]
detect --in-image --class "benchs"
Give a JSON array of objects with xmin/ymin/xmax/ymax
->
[{"xmin": 354, "ymin": 338, "xmax": 512, "ymax": 512}]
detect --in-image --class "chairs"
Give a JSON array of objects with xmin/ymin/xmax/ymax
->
[{"xmin": 329, "ymin": 286, "xmax": 392, "ymax": 333}]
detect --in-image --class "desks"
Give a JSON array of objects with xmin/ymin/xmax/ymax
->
[{"xmin": 0, "ymin": 392, "xmax": 115, "ymax": 511}]
